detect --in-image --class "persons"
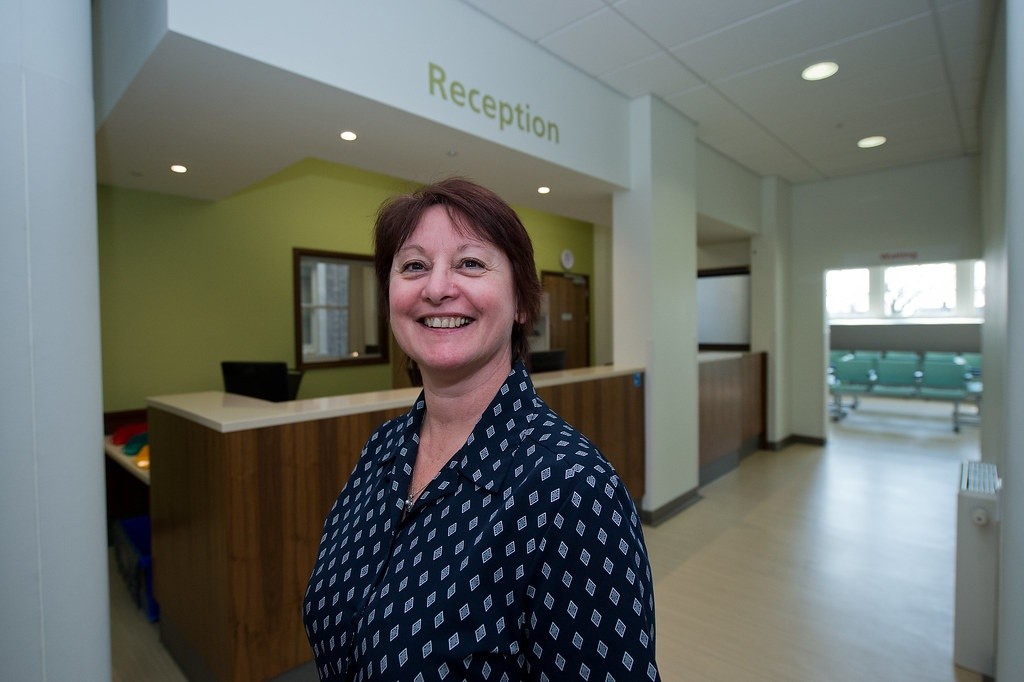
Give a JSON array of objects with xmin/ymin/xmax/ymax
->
[{"xmin": 305, "ymin": 180, "xmax": 662, "ymax": 682}]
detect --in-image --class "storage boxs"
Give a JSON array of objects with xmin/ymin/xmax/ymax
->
[{"xmin": 116, "ymin": 513, "xmax": 158, "ymax": 620}]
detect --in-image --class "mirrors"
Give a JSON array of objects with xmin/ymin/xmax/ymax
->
[{"xmin": 293, "ymin": 248, "xmax": 391, "ymax": 371}]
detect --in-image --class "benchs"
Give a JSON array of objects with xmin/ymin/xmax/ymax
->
[{"xmin": 828, "ymin": 350, "xmax": 982, "ymax": 433}]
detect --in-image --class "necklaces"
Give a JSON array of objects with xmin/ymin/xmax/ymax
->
[{"xmin": 405, "ymin": 468, "xmax": 429, "ymax": 512}]
